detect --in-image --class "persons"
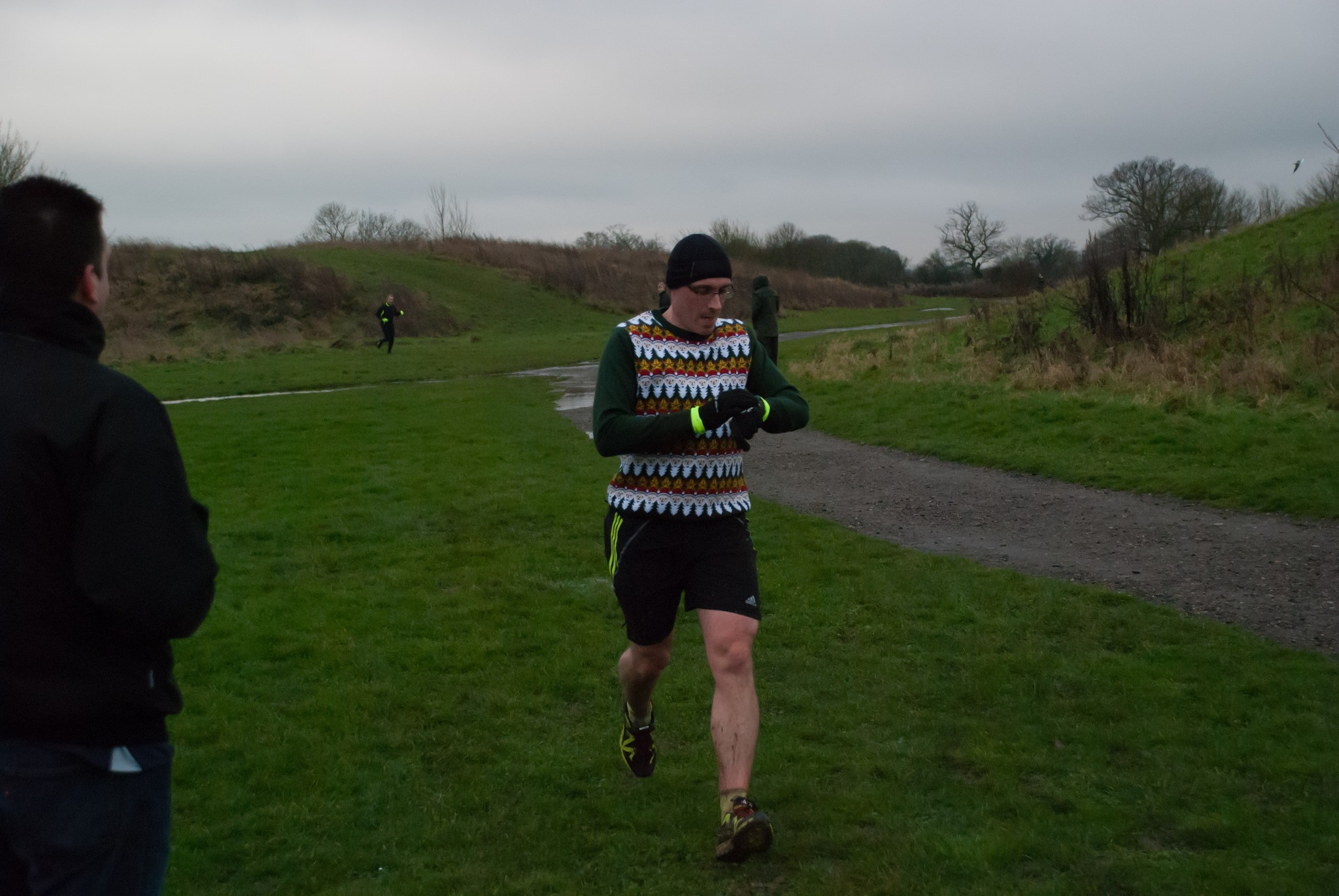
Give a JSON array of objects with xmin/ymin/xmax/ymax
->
[
  {"xmin": 0, "ymin": 171, "xmax": 221, "ymax": 895},
  {"xmin": 748, "ymin": 273, "xmax": 782, "ymax": 365},
  {"xmin": 374, "ymin": 294, "xmax": 405, "ymax": 355},
  {"xmin": 655, "ymin": 282, "xmax": 669, "ymax": 310},
  {"xmin": 589, "ymin": 230, "xmax": 812, "ymax": 866}
]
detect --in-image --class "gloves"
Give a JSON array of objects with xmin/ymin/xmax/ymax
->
[
  {"xmin": 700, "ymin": 389, "xmax": 757, "ymax": 430},
  {"xmin": 400, "ymin": 310, "xmax": 404, "ymax": 315},
  {"xmin": 729, "ymin": 400, "xmax": 766, "ymax": 452},
  {"xmin": 382, "ymin": 318, "xmax": 388, "ymax": 323}
]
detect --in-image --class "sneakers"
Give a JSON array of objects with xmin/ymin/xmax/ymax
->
[
  {"xmin": 619, "ymin": 695, "xmax": 658, "ymax": 778},
  {"xmin": 377, "ymin": 339, "xmax": 382, "ymax": 348},
  {"xmin": 387, "ymin": 347, "xmax": 391, "ymax": 355},
  {"xmin": 713, "ymin": 796, "xmax": 773, "ymax": 864}
]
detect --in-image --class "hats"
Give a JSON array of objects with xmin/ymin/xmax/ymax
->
[
  {"xmin": 752, "ymin": 275, "xmax": 769, "ymax": 291},
  {"xmin": 666, "ymin": 232, "xmax": 732, "ymax": 290}
]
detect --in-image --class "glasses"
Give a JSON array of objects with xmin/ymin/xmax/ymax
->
[{"xmin": 684, "ymin": 282, "xmax": 735, "ymax": 300}]
